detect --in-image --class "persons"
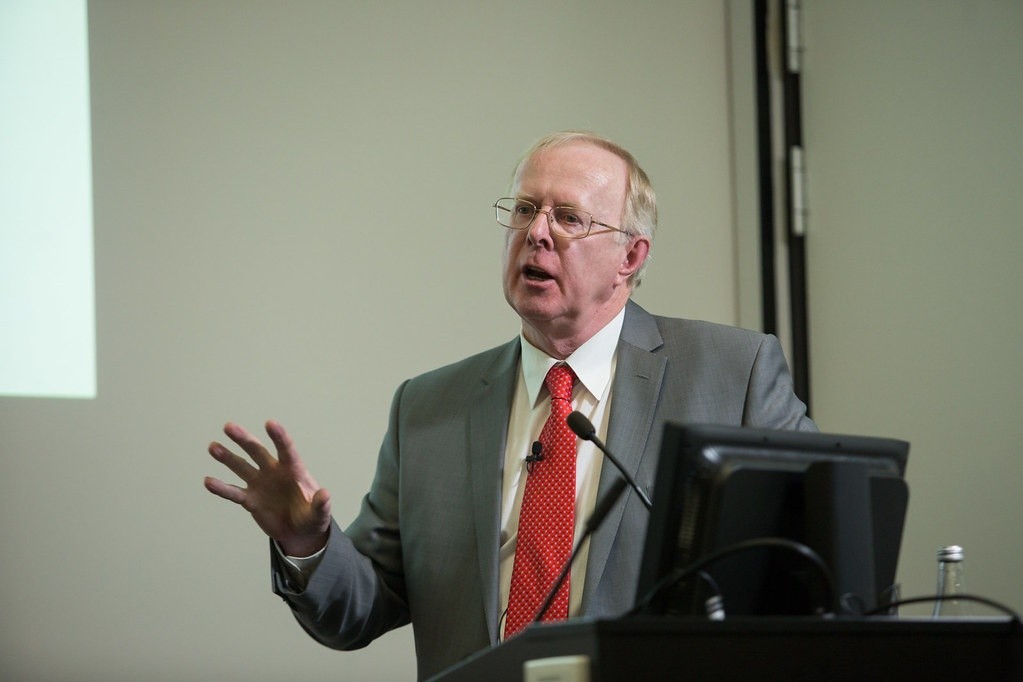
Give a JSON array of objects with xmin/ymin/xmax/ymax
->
[{"xmin": 204, "ymin": 132, "xmax": 820, "ymax": 682}]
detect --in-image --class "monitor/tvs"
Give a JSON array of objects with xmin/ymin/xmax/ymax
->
[{"xmin": 634, "ymin": 418, "xmax": 911, "ymax": 617}]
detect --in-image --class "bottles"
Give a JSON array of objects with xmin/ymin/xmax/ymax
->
[{"xmin": 931, "ymin": 545, "xmax": 973, "ymax": 616}]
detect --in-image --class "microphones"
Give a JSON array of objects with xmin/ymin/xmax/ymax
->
[
  {"xmin": 531, "ymin": 475, "xmax": 627, "ymax": 622},
  {"xmin": 566, "ymin": 411, "xmax": 652, "ymax": 508},
  {"xmin": 525, "ymin": 441, "xmax": 544, "ymax": 475}
]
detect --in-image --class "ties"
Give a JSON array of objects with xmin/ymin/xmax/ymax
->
[{"xmin": 504, "ymin": 362, "xmax": 576, "ymax": 642}]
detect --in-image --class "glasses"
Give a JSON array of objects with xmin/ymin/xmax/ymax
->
[{"xmin": 493, "ymin": 198, "xmax": 638, "ymax": 239}]
